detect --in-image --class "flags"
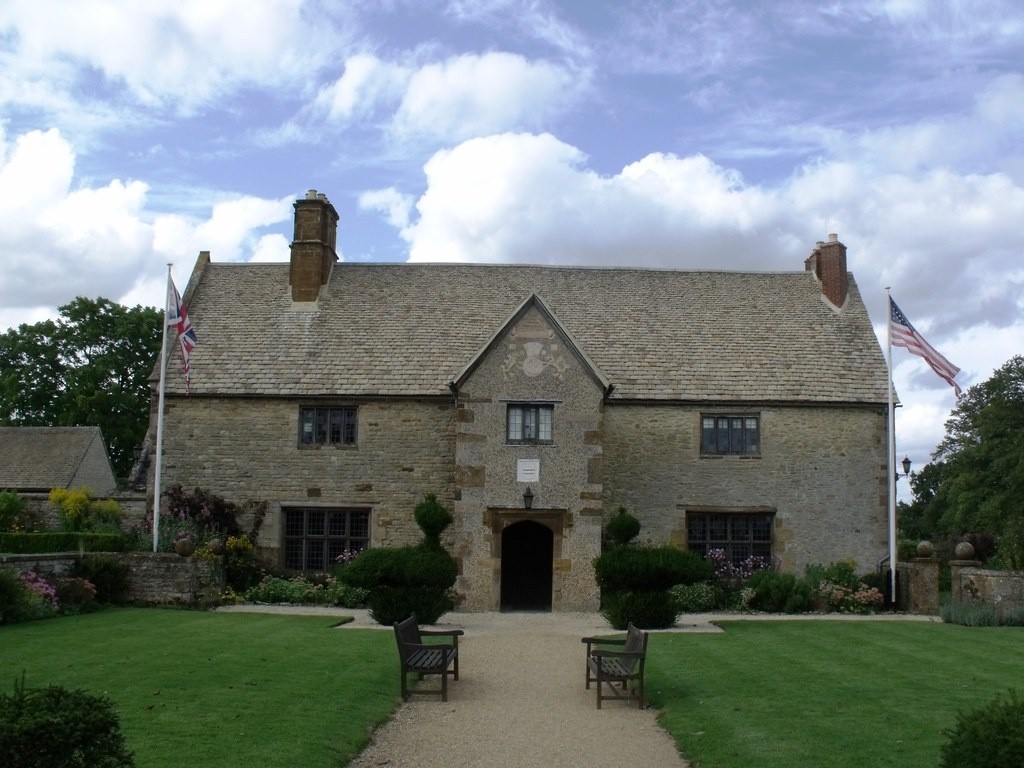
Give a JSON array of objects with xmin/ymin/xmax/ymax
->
[
  {"xmin": 888, "ymin": 295, "xmax": 962, "ymax": 399},
  {"xmin": 167, "ymin": 276, "xmax": 197, "ymax": 396}
]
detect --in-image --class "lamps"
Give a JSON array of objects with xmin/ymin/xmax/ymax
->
[{"xmin": 522, "ymin": 485, "xmax": 535, "ymax": 508}]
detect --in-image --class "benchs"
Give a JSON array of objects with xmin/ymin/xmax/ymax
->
[
  {"xmin": 581, "ymin": 622, "xmax": 648, "ymax": 711},
  {"xmin": 394, "ymin": 611, "xmax": 463, "ymax": 701}
]
